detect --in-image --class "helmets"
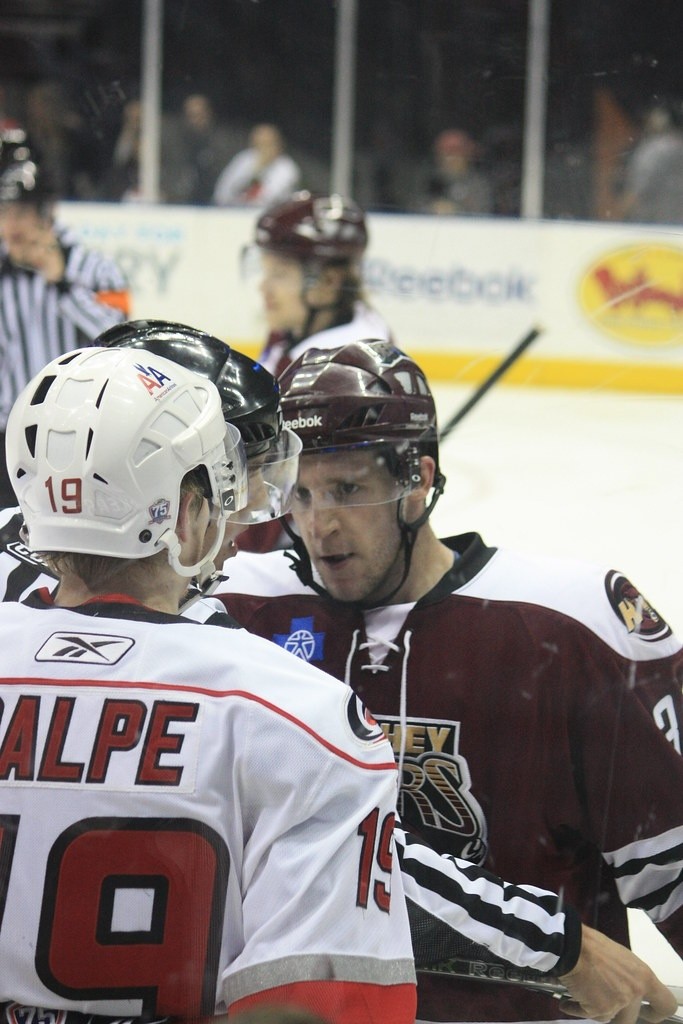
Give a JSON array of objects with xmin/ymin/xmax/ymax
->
[
  {"xmin": 86, "ymin": 318, "xmax": 281, "ymax": 420},
  {"xmin": 0, "ymin": 134, "xmax": 56, "ymax": 199},
  {"xmin": 3, "ymin": 344, "xmax": 226, "ymax": 559},
  {"xmin": 254, "ymin": 193, "xmax": 369, "ymax": 260},
  {"xmin": 275, "ymin": 339, "xmax": 439, "ymax": 452}
]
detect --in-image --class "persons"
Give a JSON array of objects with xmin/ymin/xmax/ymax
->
[
  {"xmin": 0, "ymin": 348, "xmax": 419, "ymax": 1024},
  {"xmin": 78, "ymin": 99, "xmax": 141, "ymax": 204},
  {"xmin": 1, "ymin": 165, "xmax": 130, "ymax": 512},
  {"xmin": 162, "ymin": 95, "xmax": 301, "ymax": 209},
  {"xmin": 234, "ymin": 191, "xmax": 393, "ymax": 554},
  {"xmin": 414, "ymin": 132, "xmax": 491, "ymax": 215},
  {"xmin": 1, "ymin": 318, "xmax": 678, "ymax": 1024},
  {"xmin": 218, "ymin": 337, "xmax": 683, "ymax": 1023},
  {"xmin": 621, "ymin": 94, "xmax": 683, "ymax": 223}
]
